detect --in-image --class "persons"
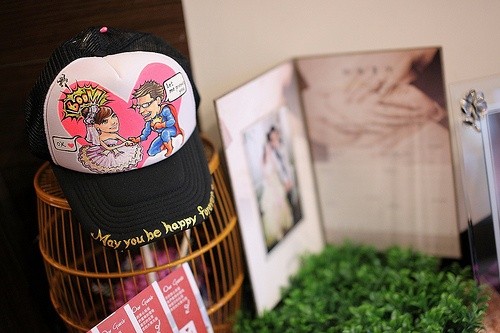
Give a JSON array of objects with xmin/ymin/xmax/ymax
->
[
  {"xmin": 296, "ymin": 46, "xmax": 462, "ymax": 261},
  {"xmin": 258, "ymin": 143, "xmax": 295, "ymax": 250},
  {"xmin": 267, "ymin": 125, "xmax": 298, "ymax": 223}
]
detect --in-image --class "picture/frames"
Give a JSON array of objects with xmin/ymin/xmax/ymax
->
[{"xmin": 213, "ymin": 45, "xmax": 463, "ymax": 321}]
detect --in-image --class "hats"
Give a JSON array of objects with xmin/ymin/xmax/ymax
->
[{"xmin": 24, "ymin": 25, "xmax": 216, "ymax": 250}]
